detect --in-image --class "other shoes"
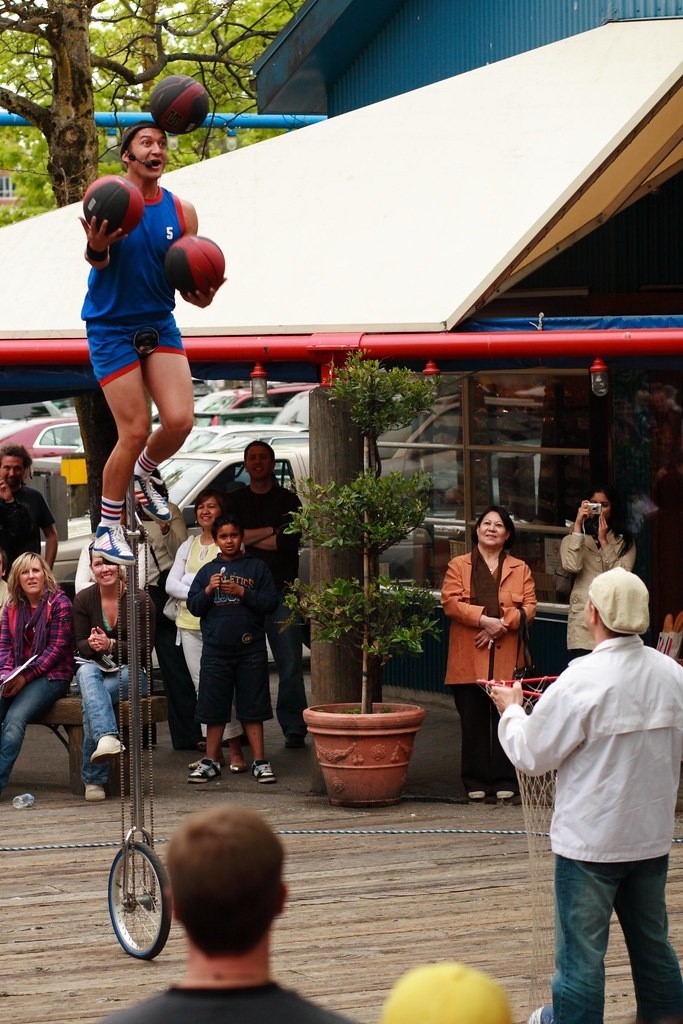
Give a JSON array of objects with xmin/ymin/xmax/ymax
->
[
  {"xmin": 188, "ymin": 760, "xmax": 226, "ymax": 769},
  {"xmin": 285, "ymin": 734, "xmax": 305, "ymax": 748},
  {"xmin": 468, "ymin": 791, "xmax": 514, "ymax": 800},
  {"xmin": 229, "ymin": 758, "xmax": 246, "ymax": 773}
]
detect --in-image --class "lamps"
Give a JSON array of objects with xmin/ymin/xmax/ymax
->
[
  {"xmin": 168, "ymin": 134, "xmax": 180, "ymax": 150},
  {"xmin": 106, "ymin": 126, "xmax": 118, "ymax": 150},
  {"xmin": 422, "ymin": 361, "xmax": 441, "ymax": 398},
  {"xmin": 226, "ymin": 128, "xmax": 238, "ymax": 152},
  {"xmin": 251, "ymin": 363, "xmax": 269, "ymax": 408},
  {"xmin": 590, "ymin": 359, "xmax": 609, "ymax": 398}
]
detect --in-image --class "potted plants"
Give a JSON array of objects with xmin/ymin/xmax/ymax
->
[{"xmin": 273, "ymin": 349, "xmax": 443, "ymax": 809}]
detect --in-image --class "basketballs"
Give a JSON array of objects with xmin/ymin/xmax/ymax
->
[
  {"xmin": 82, "ymin": 173, "xmax": 147, "ymax": 228},
  {"xmin": 149, "ymin": 76, "xmax": 210, "ymax": 134},
  {"xmin": 166, "ymin": 233, "xmax": 226, "ymax": 294}
]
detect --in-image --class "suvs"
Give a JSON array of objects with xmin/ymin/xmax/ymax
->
[{"xmin": 189, "ymin": 382, "xmax": 318, "ymax": 427}]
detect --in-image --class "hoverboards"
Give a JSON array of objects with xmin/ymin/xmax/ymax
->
[{"xmin": 81, "ymin": 470, "xmax": 186, "ymax": 961}]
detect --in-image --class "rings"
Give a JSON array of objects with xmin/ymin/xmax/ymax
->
[{"xmin": 490, "ymin": 640, "xmax": 494, "ymax": 642}]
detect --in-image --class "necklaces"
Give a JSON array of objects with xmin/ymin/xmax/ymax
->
[
  {"xmin": 487, "ymin": 559, "xmax": 498, "ymax": 571},
  {"xmin": 182, "ymin": 972, "xmax": 269, "ymax": 982}
]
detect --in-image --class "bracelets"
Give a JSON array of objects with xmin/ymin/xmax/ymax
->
[
  {"xmin": 86, "ymin": 242, "xmax": 108, "ymax": 263},
  {"xmin": 108, "ymin": 639, "xmax": 118, "ymax": 652}
]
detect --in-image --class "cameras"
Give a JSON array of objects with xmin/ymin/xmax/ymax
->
[{"xmin": 586, "ymin": 503, "xmax": 602, "ymax": 514}]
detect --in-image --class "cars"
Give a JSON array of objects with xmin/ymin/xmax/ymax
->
[
  {"xmin": 0, "ymin": 379, "xmax": 312, "ymax": 478},
  {"xmin": 41, "ymin": 444, "xmax": 314, "ymax": 596},
  {"xmin": 373, "ymin": 391, "xmax": 548, "ymax": 506}
]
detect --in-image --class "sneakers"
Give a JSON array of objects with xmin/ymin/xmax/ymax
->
[
  {"xmin": 85, "ymin": 783, "xmax": 107, "ymax": 802},
  {"xmin": 250, "ymin": 759, "xmax": 278, "ymax": 783},
  {"xmin": 89, "ymin": 736, "xmax": 127, "ymax": 764},
  {"xmin": 187, "ymin": 758, "xmax": 222, "ymax": 784},
  {"xmin": 92, "ymin": 524, "xmax": 137, "ymax": 566},
  {"xmin": 132, "ymin": 476, "xmax": 172, "ymax": 522}
]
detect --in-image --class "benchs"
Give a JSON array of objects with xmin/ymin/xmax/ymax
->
[{"xmin": 28, "ymin": 686, "xmax": 173, "ymax": 798}]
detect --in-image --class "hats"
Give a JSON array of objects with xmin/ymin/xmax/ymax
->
[{"xmin": 587, "ymin": 567, "xmax": 651, "ymax": 636}]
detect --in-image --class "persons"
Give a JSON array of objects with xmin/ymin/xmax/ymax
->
[
  {"xmin": 74, "ymin": 542, "xmax": 154, "ymax": 800},
  {"xmin": 95, "ymin": 802, "xmax": 511, "ymax": 1024},
  {"xmin": 625, "ymin": 380, "xmax": 683, "ymax": 568},
  {"xmin": 221, "ymin": 439, "xmax": 307, "ymax": 751},
  {"xmin": 188, "ymin": 513, "xmax": 277, "ymax": 783},
  {"xmin": 561, "ymin": 483, "xmax": 637, "ymax": 664},
  {"xmin": 74, "ymin": 468, "xmax": 206, "ymax": 751},
  {"xmin": 79, "ymin": 122, "xmax": 228, "ymax": 565},
  {"xmin": 490, "ymin": 567, "xmax": 683, "ymax": 1024},
  {"xmin": 0, "ymin": 443, "xmax": 59, "ymax": 614},
  {"xmin": 166, "ymin": 490, "xmax": 246, "ymax": 773},
  {"xmin": 440, "ymin": 506, "xmax": 537, "ymax": 799},
  {"xmin": 0, "ymin": 552, "xmax": 73, "ymax": 796}
]
